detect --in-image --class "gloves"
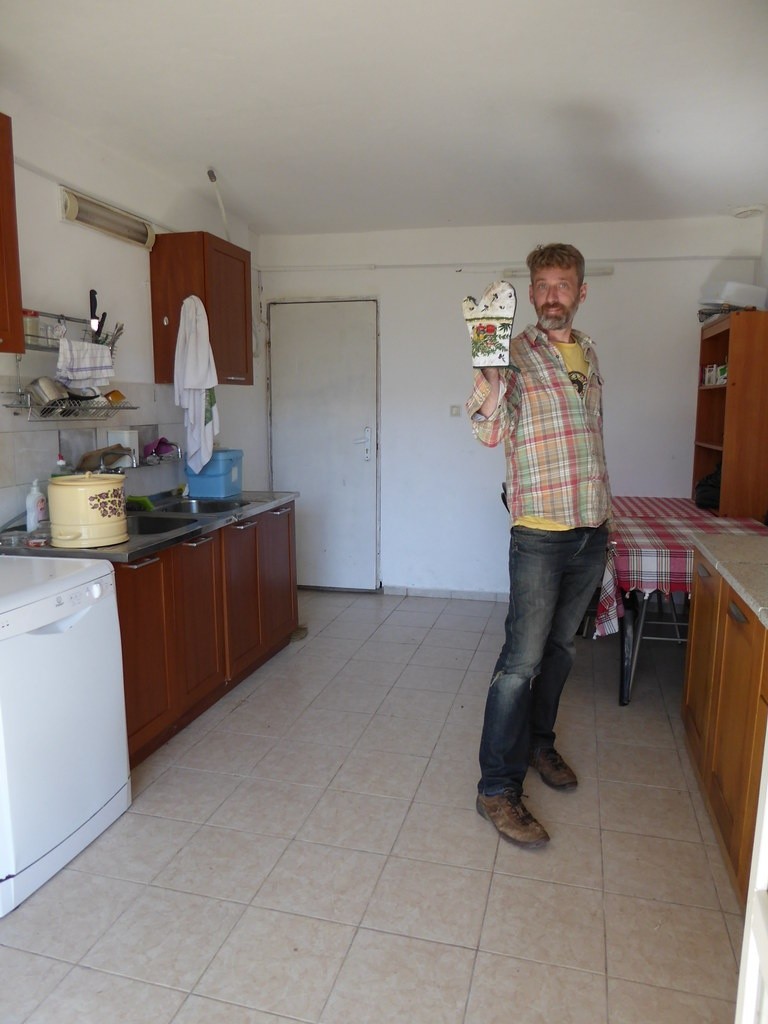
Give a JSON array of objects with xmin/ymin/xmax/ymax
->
[{"xmin": 460, "ymin": 281, "xmax": 518, "ymax": 368}]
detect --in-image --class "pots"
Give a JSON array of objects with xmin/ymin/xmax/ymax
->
[{"xmin": 47, "ymin": 471, "xmax": 129, "ymax": 548}]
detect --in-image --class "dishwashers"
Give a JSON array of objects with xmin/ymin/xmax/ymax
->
[{"xmin": 0, "ymin": 553, "xmax": 133, "ymax": 921}]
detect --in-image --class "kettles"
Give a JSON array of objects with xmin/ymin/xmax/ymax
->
[{"xmin": 26, "ymin": 376, "xmax": 68, "ymax": 417}]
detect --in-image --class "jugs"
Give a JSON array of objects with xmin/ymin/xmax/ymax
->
[{"xmin": 81, "ymin": 386, "xmax": 109, "ymax": 417}]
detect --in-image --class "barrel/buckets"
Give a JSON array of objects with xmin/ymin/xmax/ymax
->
[{"xmin": 183, "ymin": 449, "xmax": 244, "ymax": 498}]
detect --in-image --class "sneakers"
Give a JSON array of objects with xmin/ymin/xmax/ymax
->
[
  {"xmin": 475, "ymin": 783, "xmax": 552, "ymax": 849},
  {"xmin": 527, "ymin": 741, "xmax": 578, "ymax": 790}
]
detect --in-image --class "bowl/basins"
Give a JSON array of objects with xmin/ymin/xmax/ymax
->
[
  {"xmin": 30, "ymin": 521, "xmax": 51, "ymax": 542},
  {"xmin": 1, "ymin": 531, "xmax": 28, "ymax": 546}
]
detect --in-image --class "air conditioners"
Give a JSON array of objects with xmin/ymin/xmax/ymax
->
[{"xmin": 61, "ymin": 186, "xmax": 155, "ymax": 249}]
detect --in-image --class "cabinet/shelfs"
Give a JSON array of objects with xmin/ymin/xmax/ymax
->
[
  {"xmin": 684, "ymin": 546, "xmax": 767, "ymax": 919},
  {"xmin": 0, "ymin": 112, "xmax": 26, "ymax": 354},
  {"xmin": 111, "ymin": 500, "xmax": 299, "ymax": 769},
  {"xmin": 690, "ymin": 310, "xmax": 767, "ymax": 522},
  {"xmin": 150, "ymin": 231, "xmax": 255, "ymax": 386}
]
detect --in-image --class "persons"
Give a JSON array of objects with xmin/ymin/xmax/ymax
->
[{"xmin": 463, "ymin": 245, "xmax": 614, "ymax": 851}]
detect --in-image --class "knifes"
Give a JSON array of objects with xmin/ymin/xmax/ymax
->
[{"xmin": 89, "ymin": 289, "xmax": 100, "ymax": 332}]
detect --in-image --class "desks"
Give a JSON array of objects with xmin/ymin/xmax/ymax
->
[{"xmin": 592, "ymin": 495, "xmax": 768, "ymax": 705}]
